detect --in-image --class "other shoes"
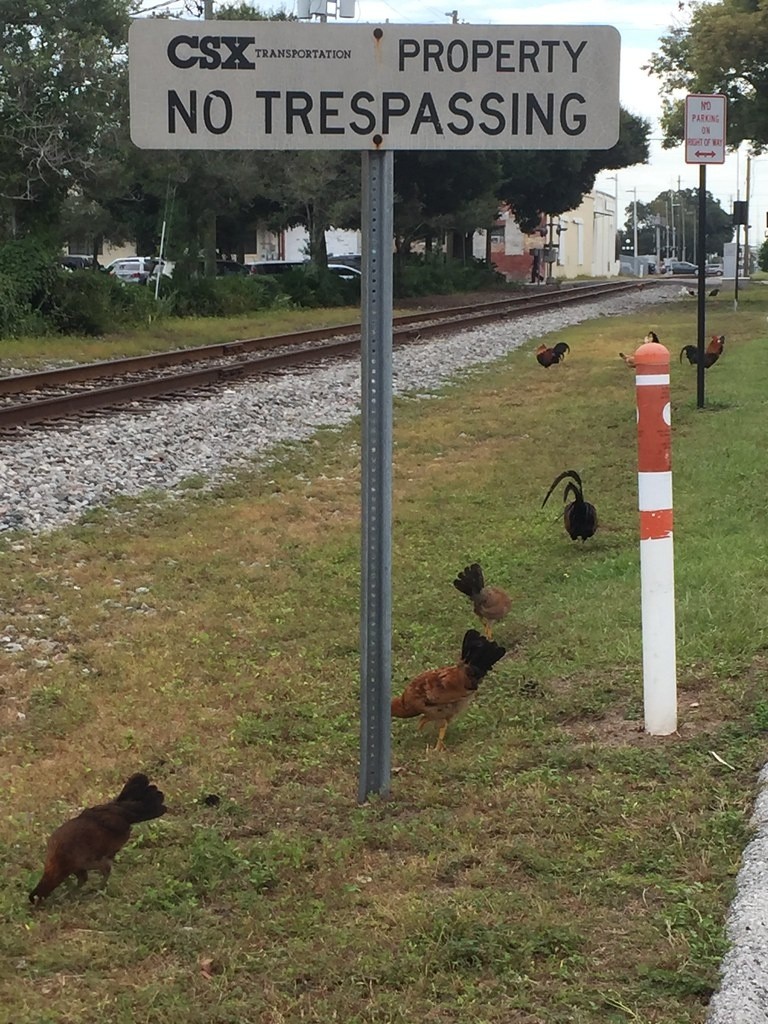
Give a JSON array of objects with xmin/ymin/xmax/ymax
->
[
  {"xmin": 541, "ymin": 277, "xmax": 543, "ymax": 282},
  {"xmin": 529, "ymin": 281, "xmax": 535, "ymax": 283}
]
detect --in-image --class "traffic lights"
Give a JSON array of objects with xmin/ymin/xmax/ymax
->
[
  {"xmin": 536, "ymin": 227, "xmax": 548, "ymax": 237},
  {"xmin": 556, "ymin": 227, "xmax": 567, "ymax": 236}
]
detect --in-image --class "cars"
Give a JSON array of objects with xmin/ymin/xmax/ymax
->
[
  {"xmin": 110, "ymin": 262, "xmax": 151, "ymax": 283},
  {"xmin": 660, "ymin": 262, "xmax": 699, "ymax": 275},
  {"xmin": 147, "ymin": 259, "xmax": 361, "ymax": 284},
  {"xmin": 106, "ymin": 256, "xmax": 164, "ymax": 274},
  {"xmin": 705, "ymin": 264, "xmax": 723, "ymax": 276},
  {"xmin": 63, "ymin": 255, "xmax": 104, "ymax": 272}
]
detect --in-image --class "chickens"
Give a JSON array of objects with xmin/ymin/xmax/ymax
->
[
  {"xmin": 540, "ymin": 469, "xmax": 599, "ymax": 544},
  {"xmin": 536, "ymin": 342, "xmax": 570, "ymax": 368},
  {"xmin": 391, "ymin": 563, "xmax": 511, "ymax": 753},
  {"xmin": 617, "ymin": 331, "xmax": 659, "ymax": 367},
  {"xmin": 680, "ymin": 336, "xmax": 725, "ymax": 369},
  {"xmin": 26, "ymin": 772, "xmax": 168, "ymax": 907}
]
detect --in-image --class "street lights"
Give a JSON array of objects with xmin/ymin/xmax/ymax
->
[
  {"xmin": 686, "ymin": 210, "xmax": 696, "ymax": 265},
  {"xmin": 672, "ymin": 204, "xmax": 680, "ymax": 257},
  {"xmin": 626, "ymin": 187, "xmax": 637, "ymax": 256},
  {"xmin": 607, "ymin": 174, "xmax": 617, "ymax": 198}
]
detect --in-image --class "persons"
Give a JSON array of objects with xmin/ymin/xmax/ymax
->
[{"xmin": 528, "ymin": 248, "xmax": 543, "ymax": 283}]
